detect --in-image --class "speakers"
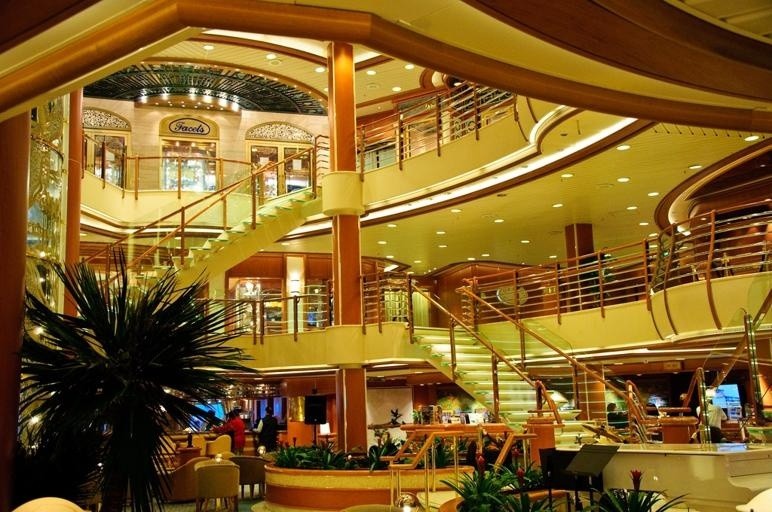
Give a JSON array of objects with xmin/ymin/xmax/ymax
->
[{"xmin": 304, "ymin": 395, "xmax": 328, "ymax": 425}]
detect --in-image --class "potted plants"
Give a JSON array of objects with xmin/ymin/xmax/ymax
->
[
  {"xmin": 264, "ymin": 437, "xmax": 475, "ymax": 512},
  {"xmin": 437, "ymin": 446, "xmax": 696, "ymax": 512}
]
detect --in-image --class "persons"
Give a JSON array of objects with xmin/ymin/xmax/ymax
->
[
  {"xmin": 607, "ymin": 401, "xmax": 628, "ymax": 430},
  {"xmin": 701, "ymin": 397, "xmax": 728, "ymax": 442},
  {"xmin": 203, "ymin": 410, "xmax": 224, "ymax": 431},
  {"xmin": 210, "ymin": 408, "xmax": 247, "ymax": 456},
  {"xmin": 251, "ymin": 404, "xmax": 278, "ymax": 454}
]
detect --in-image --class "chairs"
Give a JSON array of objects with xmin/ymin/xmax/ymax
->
[{"xmin": 156, "ymin": 435, "xmax": 271, "ymax": 512}]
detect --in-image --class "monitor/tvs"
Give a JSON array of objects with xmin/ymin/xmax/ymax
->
[{"xmin": 708, "ymin": 383, "xmax": 743, "ymax": 411}]
